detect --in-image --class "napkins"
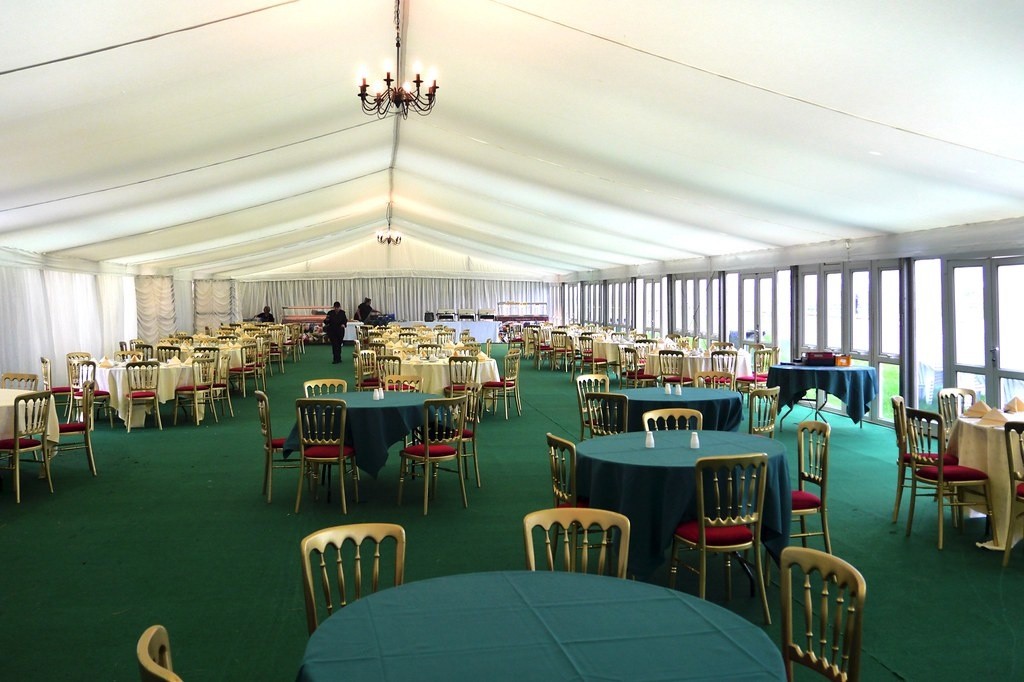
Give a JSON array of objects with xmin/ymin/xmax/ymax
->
[
  {"xmin": 962, "ymin": 401, "xmax": 992, "ymax": 418},
  {"xmin": 978, "ymin": 406, "xmax": 1007, "ymax": 428},
  {"xmin": 1003, "ymin": 396, "xmax": 1024, "ymax": 413}
]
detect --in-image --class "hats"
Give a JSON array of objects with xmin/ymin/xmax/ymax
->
[{"xmin": 334, "ymin": 302, "xmax": 341, "ymax": 307}]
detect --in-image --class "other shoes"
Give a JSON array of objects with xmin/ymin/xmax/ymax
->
[
  {"xmin": 323, "ymin": 326, "xmax": 327, "ymax": 333},
  {"xmin": 332, "ymin": 360, "xmax": 342, "ymax": 364}
]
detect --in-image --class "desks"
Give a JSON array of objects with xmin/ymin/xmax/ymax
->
[
  {"xmin": 592, "ymin": 338, "xmax": 676, "ymax": 379},
  {"xmin": 378, "ymin": 355, "xmax": 501, "ymax": 420},
  {"xmin": 944, "ymin": 410, "xmax": 1024, "ymax": 551},
  {"xmin": 764, "ymin": 364, "xmax": 879, "ymax": 432},
  {"xmin": 282, "ymin": 389, "xmax": 460, "ymax": 505},
  {"xmin": 643, "ymin": 348, "xmax": 753, "ymax": 386},
  {"xmin": 296, "ymin": 569, "xmax": 787, "ymax": 682},
  {"xmin": 151, "ymin": 340, "xmax": 247, "ymax": 396},
  {"xmin": 388, "ymin": 319, "xmax": 502, "ymax": 345},
  {"xmin": 74, "ymin": 359, "xmax": 208, "ymax": 432},
  {"xmin": 342, "ymin": 320, "xmax": 365, "ymax": 341},
  {"xmin": 0, "ymin": 388, "xmax": 60, "ymax": 479},
  {"xmin": 597, "ymin": 385, "xmax": 745, "ymax": 432},
  {"xmin": 570, "ymin": 425, "xmax": 793, "ymax": 601}
]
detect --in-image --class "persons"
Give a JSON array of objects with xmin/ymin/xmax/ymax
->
[
  {"xmin": 254, "ymin": 306, "xmax": 275, "ymax": 323},
  {"xmin": 323, "ymin": 302, "xmax": 348, "ymax": 365},
  {"xmin": 354, "ymin": 297, "xmax": 383, "ymax": 325},
  {"xmin": 311, "ymin": 310, "xmax": 326, "ymax": 316}
]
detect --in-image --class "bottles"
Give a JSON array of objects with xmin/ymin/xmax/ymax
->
[
  {"xmin": 690, "ymin": 432, "xmax": 700, "ymax": 448},
  {"xmin": 373, "ymin": 388, "xmax": 379, "ymax": 400},
  {"xmin": 646, "ymin": 431, "xmax": 655, "ymax": 448},
  {"xmin": 378, "ymin": 388, "xmax": 384, "ymax": 399},
  {"xmin": 675, "ymin": 385, "xmax": 682, "ymax": 395},
  {"xmin": 665, "ymin": 384, "xmax": 671, "ymax": 394}
]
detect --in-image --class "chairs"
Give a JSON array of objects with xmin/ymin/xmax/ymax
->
[{"xmin": 0, "ymin": 319, "xmax": 1024, "ymax": 682}]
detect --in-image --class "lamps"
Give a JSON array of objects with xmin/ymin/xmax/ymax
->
[
  {"xmin": 377, "ymin": 222, "xmax": 402, "ymax": 246},
  {"xmin": 357, "ymin": 1, "xmax": 439, "ymax": 119}
]
